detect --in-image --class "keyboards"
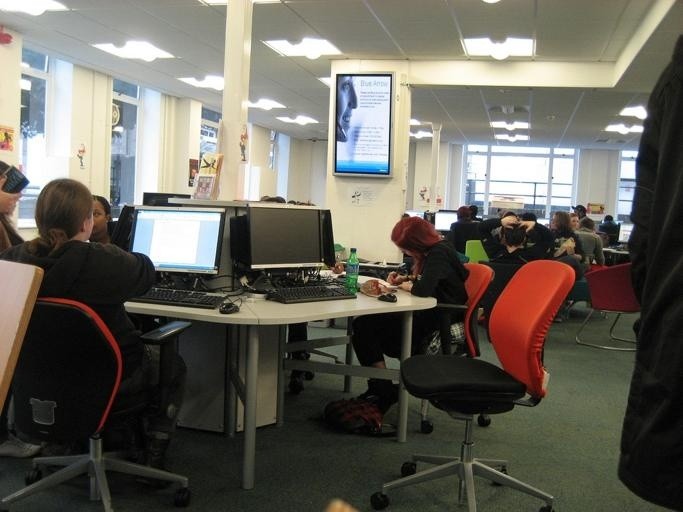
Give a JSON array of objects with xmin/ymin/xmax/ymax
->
[
  {"xmin": 274, "ymin": 286, "xmax": 357, "ymax": 304},
  {"xmin": 336, "ymin": 278, "xmax": 361, "ymax": 292},
  {"xmin": 127, "ymin": 286, "xmax": 224, "ymax": 310}
]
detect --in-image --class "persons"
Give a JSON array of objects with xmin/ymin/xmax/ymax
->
[
  {"xmin": 78, "ymin": 143, "xmax": 86, "ymax": 166},
  {"xmin": 201, "ymin": 159, "xmax": 216, "ymax": 170},
  {"xmin": 240, "ymin": 124, "xmax": 247, "ymax": 161},
  {"xmin": 353, "ymin": 217, "xmax": 468, "ymax": 400},
  {"xmin": 0, "ymin": 160, "xmax": 26, "ymax": 252},
  {"xmin": 445, "ymin": 206, "xmax": 618, "ymax": 323},
  {"xmin": 1, "ymin": 179, "xmax": 186, "ymax": 487},
  {"xmin": 337, "ymin": 75, "xmax": 357, "ymax": 142},
  {"xmin": 616, "ymin": 35, "xmax": 682, "ymax": 511},
  {"xmin": 3, "ymin": 132, "xmax": 11, "ymax": 146},
  {"xmin": 89, "ymin": 195, "xmax": 118, "ymax": 244}
]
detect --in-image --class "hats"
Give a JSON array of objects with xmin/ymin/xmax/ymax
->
[{"xmin": 571, "ymin": 205, "xmax": 586, "ymax": 214}]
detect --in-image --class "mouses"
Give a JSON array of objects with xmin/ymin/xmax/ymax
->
[
  {"xmin": 378, "ymin": 293, "xmax": 397, "ymax": 303},
  {"xmin": 338, "ymin": 274, "xmax": 347, "ymax": 278},
  {"xmin": 219, "ymin": 303, "xmax": 239, "ymax": 314}
]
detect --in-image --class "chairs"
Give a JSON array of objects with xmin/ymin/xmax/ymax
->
[
  {"xmin": 1, "ymin": 294, "xmax": 197, "ymax": 511},
  {"xmin": 367, "ymin": 258, "xmax": 578, "ymax": 511},
  {"xmin": 412, "ymin": 261, "xmax": 499, "ymax": 435},
  {"xmin": 573, "ymin": 260, "xmax": 644, "ymax": 354},
  {"xmin": 462, "ymin": 239, "xmax": 489, "ymax": 262}
]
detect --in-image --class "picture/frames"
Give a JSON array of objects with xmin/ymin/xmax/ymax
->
[{"xmin": 330, "ymin": 71, "xmax": 397, "ymax": 181}]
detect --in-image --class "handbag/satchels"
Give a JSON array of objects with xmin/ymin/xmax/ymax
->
[{"xmin": 325, "ymin": 392, "xmax": 393, "ymax": 436}]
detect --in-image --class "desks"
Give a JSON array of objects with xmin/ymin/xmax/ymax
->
[
  {"xmin": 223, "ymin": 279, "xmax": 443, "ymax": 486},
  {"xmin": 114, "ymin": 269, "xmax": 259, "ymax": 493},
  {"xmin": 602, "ymin": 245, "xmax": 629, "ymax": 265}
]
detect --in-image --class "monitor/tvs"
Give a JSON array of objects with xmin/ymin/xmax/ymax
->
[
  {"xmin": 142, "ymin": 192, "xmax": 191, "ymax": 207},
  {"xmin": 129, "ymin": 206, "xmax": 228, "ymax": 275},
  {"xmin": 246, "ymin": 203, "xmax": 325, "ymax": 271},
  {"xmin": 403, "ymin": 210, "xmax": 426, "ymax": 221},
  {"xmin": 536, "ymin": 218, "xmax": 551, "ymax": 229},
  {"xmin": 617, "ymin": 222, "xmax": 636, "ymax": 243},
  {"xmin": 323, "ymin": 208, "xmax": 336, "ymax": 269},
  {"xmin": 433, "ymin": 211, "xmax": 459, "ymax": 232},
  {"xmin": 111, "ymin": 204, "xmax": 135, "ymax": 243}
]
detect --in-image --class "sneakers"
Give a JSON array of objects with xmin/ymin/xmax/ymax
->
[{"xmin": 0, "ymin": 433, "xmax": 41, "ymax": 459}]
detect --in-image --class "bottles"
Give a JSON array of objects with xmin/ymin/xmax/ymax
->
[{"xmin": 346, "ymin": 246, "xmax": 360, "ymax": 295}]
